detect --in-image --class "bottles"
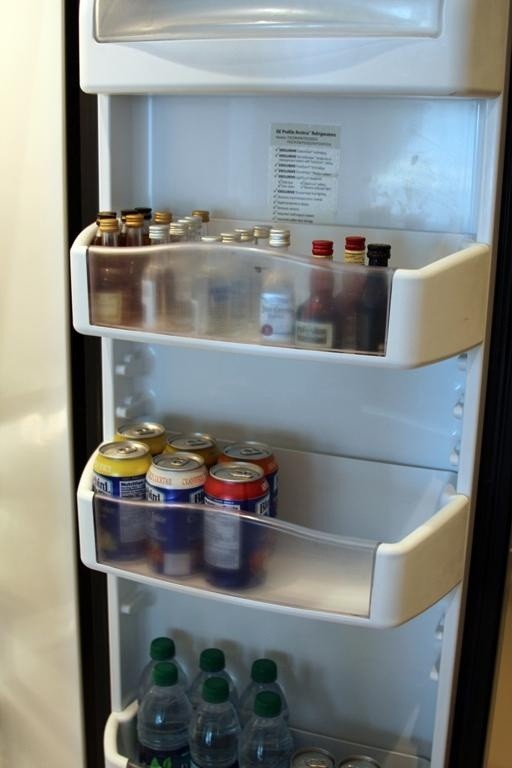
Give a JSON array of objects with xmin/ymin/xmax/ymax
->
[
  {"xmin": 170, "ymin": 209, "xmax": 209, "ymax": 241},
  {"xmin": 295, "ymin": 240, "xmax": 335, "ymax": 350},
  {"xmin": 203, "ymin": 462, "xmax": 271, "ymax": 591},
  {"xmin": 222, "ymin": 234, "xmax": 239, "ymax": 245},
  {"xmin": 94, "ymin": 441, "xmax": 152, "ymax": 561},
  {"xmin": 136, "ymin": 662, "xmax": 194, "ymax": 768},
  {"xmin": 254, "ymin": 227, "xmax": 270, "ymax": 247},
  {"xmin": 154, "ymin": 210, "xmax": 173, "ymax": 225},
  {"xmin": 145, "ymin": 451, "xmax": 207, "ymax": 578},
  {"xmin": 189, "ymin": 648, "xmax": 241, "ymax": 711},
  {"xmin": 334, "ymin": 237, "xmax": 365, "ymax": 348},
  {"xmin": 218, "ymin": 441, "xmax": 277, "ymax": 523},
  {"xmin": 337, "ymin": 756, "xmax": 380, "ymax": 768},
  {"xmin": 236, "ymin": 229, "xmax": 251, "ymax": 244},
  {"xmin": 90, "ymin": 211, "xmax": 126, "ymax": 245},
  {"xmin": 241, "ymin": 659, "xmax": 290, "ymax": 731},
  {"xmin": 114, "ymin": 422, "xmax": 167, "ymax": 457},
  {"xmin": 121, "ymin": 207, "xmax": 152, "ymax": 245},
  {"xmin": 289, "ymin": 745, "xmax": 335, "ymax": 768},
  {"xmin": 238, "ymin": 690, "xmax": 294, "ymax": 768},
  {"xmin": 356, "ymin": 244, "xmax": 391, "ymax": 357},
  {"xmin": 191, "ymin": 235, "xmax": 235, "ymax": 336},
  {"xmin": 189, "ymin": 676, "xmax": 241, "ymax": 768},
  {"xmin": 162, "ymin": 432, "xmax": 218, "ymax": 468},
  {"xmin": 141, "ymin": 225, "xmax": 175, "ymax": 330},
  {"xmin": 136, "ymin": 637, "xmax": 187, "ymax": 705},
  {"xmin": 91, "ymin": 220, "xmax": 134, "ymax": 325},
  {"xmin": 257, "ymin": 229, "xmax": 293, "ymax": 342}
]
